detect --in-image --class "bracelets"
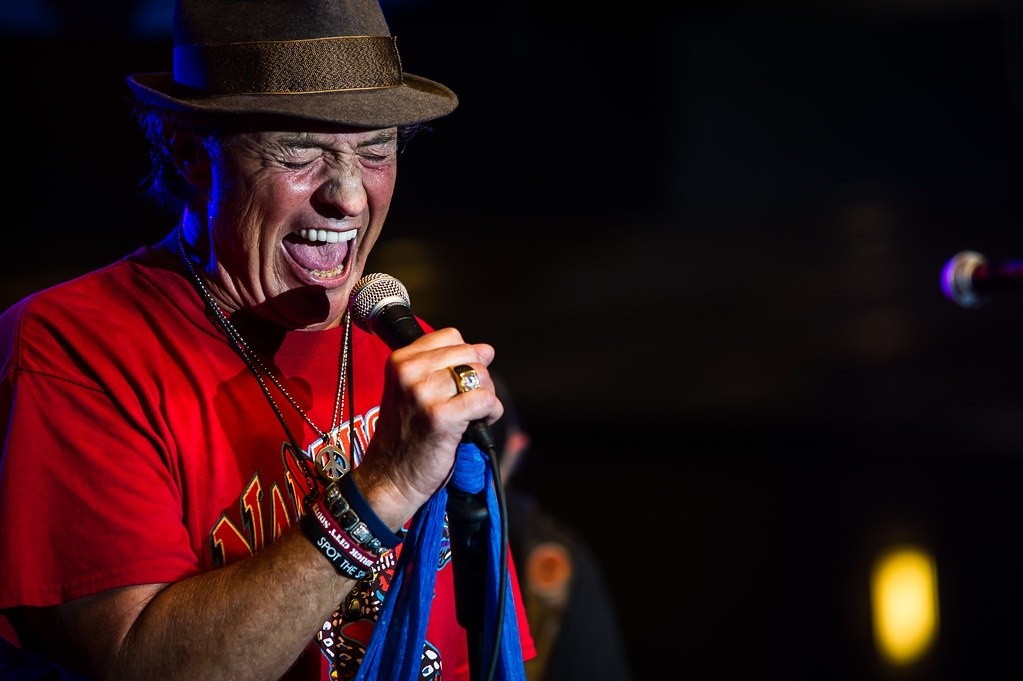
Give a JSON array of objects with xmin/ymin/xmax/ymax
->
[{"xmin": 296, "ymin": 476, "xmax": 404, "ymax": 586}]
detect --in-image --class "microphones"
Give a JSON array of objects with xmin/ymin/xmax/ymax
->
[
  {"xmin": 940, "ymin": 250, "xmax": 1023, "ymax": 308},
  {"xmin": 348, "ymin": 272, "xmax": 495, "ymax": 454}
]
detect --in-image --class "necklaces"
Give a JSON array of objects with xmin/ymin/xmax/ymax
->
[{"xmin": 190, "ymin": 268, "xmax": 355, "ymax": 496}]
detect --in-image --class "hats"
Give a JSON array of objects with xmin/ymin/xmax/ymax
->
[{"xmin": 127, "ymin": 0, "xmax": 458, "ymax": 127}]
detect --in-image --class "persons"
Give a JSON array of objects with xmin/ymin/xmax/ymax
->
[{"xmin": 0, "ymin": 0, "xmax": 505, "ymax": 681}]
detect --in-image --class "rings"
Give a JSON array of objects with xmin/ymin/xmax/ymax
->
[{"xmin": 448, "ymin": 363, "xmax": 481, "ymax": 395}]
